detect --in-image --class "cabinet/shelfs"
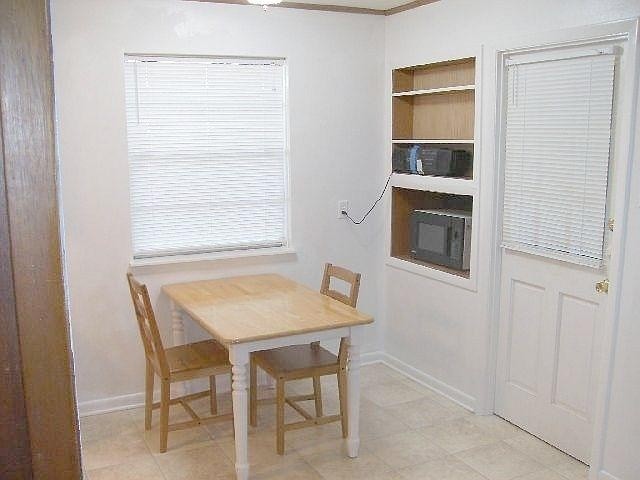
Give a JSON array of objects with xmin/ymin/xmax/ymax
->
[{"xmin": 383, "ymin": 43, "xmax": 483, "ymax": 293}]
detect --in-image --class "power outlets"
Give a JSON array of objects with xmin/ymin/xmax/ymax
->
[{"xmin": 339, "ymin": 201, "xmax": 348, "ymax": 220}]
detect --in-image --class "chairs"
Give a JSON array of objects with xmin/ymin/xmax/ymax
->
[
  {"xmin": 250, "ymin": 264, "xmax": 361, "ymax": 456},
  {"xmin": 126, "ymin": 272, "xmax": 234, "ymax": 453}
]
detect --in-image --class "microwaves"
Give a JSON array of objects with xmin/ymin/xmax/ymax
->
[{"xmin": 409, "ymin": 207, "xmax": 472, "ymax": 271}]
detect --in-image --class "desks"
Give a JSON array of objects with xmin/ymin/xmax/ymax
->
[{"xmin": 162, "ymin": 272, "xmax": 375, "ymax": 480}]
